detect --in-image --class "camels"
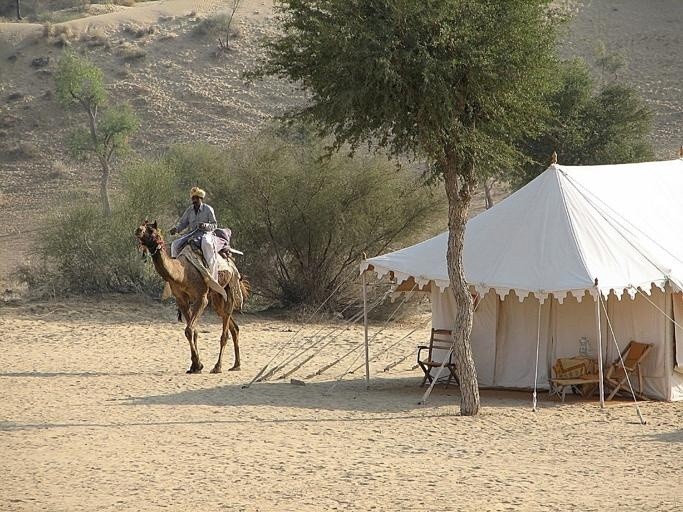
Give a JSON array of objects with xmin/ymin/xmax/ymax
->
[{"xmin": 134, "ymin": 218, "xmax": 251, "ymax": 375}]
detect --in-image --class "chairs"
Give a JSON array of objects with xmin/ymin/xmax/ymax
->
[
  {"xmin": 416, "ymin": 327, "xmax": 461, "ymax": 391},
  {"xmin": 548, "ymin": 341, "xmax": 654, "ymax": 404}
]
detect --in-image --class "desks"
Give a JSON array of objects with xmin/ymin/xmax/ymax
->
[{"xmin": 557, "ymin": 357, "xmax": 596, "ymax": 397}]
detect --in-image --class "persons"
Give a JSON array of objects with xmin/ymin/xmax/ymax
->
[{"xmin": 170, "ymin": 187, "xmax": 219, "ymax": 284}]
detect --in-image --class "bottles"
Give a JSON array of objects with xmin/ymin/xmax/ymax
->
[{"xmin": 580, "ymin": 336, "xmax": 589, "ymax": 358}]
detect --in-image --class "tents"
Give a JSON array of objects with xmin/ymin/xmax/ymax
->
[{"xmin": 359, "ymin": 150, "xmax": 683, "ymax": 408}]
